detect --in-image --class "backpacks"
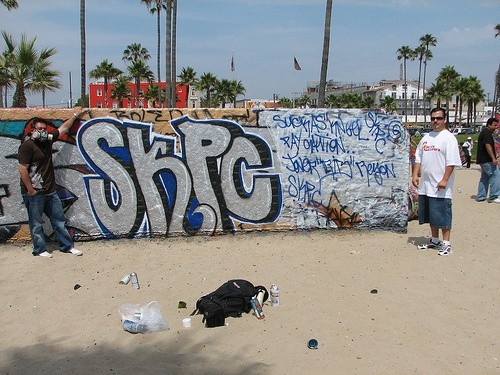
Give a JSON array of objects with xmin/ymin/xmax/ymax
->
[{"xmin": 190, "ymin": 279, "xmax": 269, "ymax": 318}]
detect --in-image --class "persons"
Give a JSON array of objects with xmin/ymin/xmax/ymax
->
[
  {"xmin": 462, "ymin": 137, "xmax": 473, "ymax": 168},
  {"xmin": 17, "ymin": 106, "xmax": 88, "ymax": 258},
  {"xmin": 476, "ymin": 118, "xmax": 500, "ymax": 203},
  {"xmin": 412, "ymin": 108, "xmax": 462, "ymax": 256}
]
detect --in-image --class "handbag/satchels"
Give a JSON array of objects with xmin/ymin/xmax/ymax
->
[
  {"xmin": 202, "ymin": 305, "xmax": 225, "ymax": 328},
  {"xmin": 117, "ymin": 301, "xmax": 170, "ymax": 334}
]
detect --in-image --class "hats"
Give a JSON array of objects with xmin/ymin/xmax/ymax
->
[{"xmin": 467, "ymin": 136, "xmax": 472, "ymax": 140}]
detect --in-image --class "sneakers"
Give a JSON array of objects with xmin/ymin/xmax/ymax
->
[
  {"xmin": 488, "ymin": 197, "xmax": 500, "ymax": 203},
  {"xmin": 417, "ymin": 238, "xmax": 441, "ymax": 250},
  {"xmin": 437, "ymin": 243, "xmax": 453, "ymax": 256}
]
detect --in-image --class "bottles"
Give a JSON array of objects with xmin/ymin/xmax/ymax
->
[
  {"xmin": 251, "ymin": 297, "xmax": 265, "ymax": 320},
  {"xmin": 119, "ymin": 274, "xmax": 130, "ymax": 285},
  {"xmin": 270, "ymin": 285, "xmax": 281, "ymax": 307},
  {"xmin": 130, "ymin": 272, "xmax": 140, "ymax": 289}
]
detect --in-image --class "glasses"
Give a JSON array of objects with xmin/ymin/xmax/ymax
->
[{"xmin": 431, "ymin": 117, "xmax": 444, "ymax": 121}]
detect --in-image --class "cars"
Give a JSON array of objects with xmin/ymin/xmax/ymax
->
[{"xmin": 419, "ymin": 128, "xmax": 477, "ymax": 137}]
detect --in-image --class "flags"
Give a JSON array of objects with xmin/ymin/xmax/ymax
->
[
  {"xmin": 294, "ymin": 57, "xmax": 301, "ymax": 70},
  {"xmin": 231, "ymin": 56, "xmax": 235, "ymax": 71}
]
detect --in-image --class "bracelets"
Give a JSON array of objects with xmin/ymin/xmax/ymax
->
[{"xmin": 74, "ymin": 113, "xmax": 79, "ymax": 119}]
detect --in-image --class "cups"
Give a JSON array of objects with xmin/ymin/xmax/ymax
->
[{"xmin": 182, "ymin": 318, "xmax": 192, "ymax": 327}]
